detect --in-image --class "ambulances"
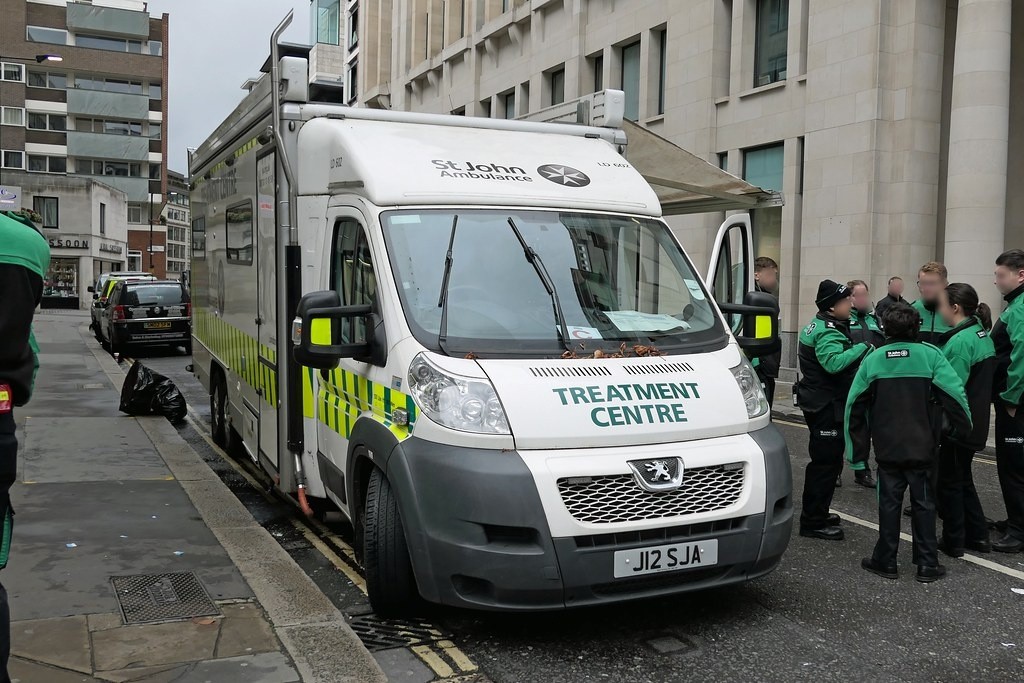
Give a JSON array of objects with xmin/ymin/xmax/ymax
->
[{"xmin": 187, "ymin": 52, "xmax": 791, "ymax": 621}]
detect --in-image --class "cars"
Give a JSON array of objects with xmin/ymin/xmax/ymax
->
[{"xmin": 88, "ymin": 270, "xmax": 192, "ymax": 361}]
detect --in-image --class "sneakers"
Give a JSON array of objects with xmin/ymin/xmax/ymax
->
[
  {"xmin": 835, "ymin": 474, "xmax": 842, "ymax": 487},
  {"xmin": 986, "ymin": 520, "xmax": 1006, "ymax": 535},
  {"xmin": 800, "ymin": 523, "xmax": 844, "ymax": 540},
  {"xmin": 903, "ymin": 505, "xmax": 912, "ymax": 515},
  {"xmin": 963, "ymin": 539, "xmax": 993, "ymax": 553},
  {"xmin": 991, "ymin": 534, "xmax": 1024, "ymax": 553},
  {"xmin": 854, "ymin": 476, "xmax": 878, "ymax": 488},
  {"xmin": 825, "ymin": 513, "xmax": 840, "ymax": 526},
  {"xmin": 936, "ymin": 537, "xmax": 965, "ymax": 557}
]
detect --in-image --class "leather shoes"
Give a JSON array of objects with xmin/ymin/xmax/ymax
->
[
  {"xmin": 862, "ymin": 557, "xmax": 899, "ymax": 579},
  {"xmin": 915, "ymin": 564, "xmax": 946, "ymax": 582}
]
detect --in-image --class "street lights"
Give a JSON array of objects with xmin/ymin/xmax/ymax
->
[{"xmin": 149, "ymin": 190, "xmax": 179, "ymax": 274}]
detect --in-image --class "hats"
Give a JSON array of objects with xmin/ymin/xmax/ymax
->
[{"xmin": 815, "ymin": 279, "xmax": 852, "ymax": 312}]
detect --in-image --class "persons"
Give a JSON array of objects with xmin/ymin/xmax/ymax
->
[
  {"xmin": 934, "ymin": 281, "xmax": 996, "ymax": 559},
  {"xmin": 902, "ymin": 261, "xmax": 956, "ymax": 516},
  {"xmin": 0, "ymin": 209, "xmax": 53, "ymax": 683},
  {"xmin": 793, "ymin": 278, "xmax": 876, "ymax": 542},
  {"xmin": 843, "ymin": 301, "xmax": 973, "ymax": 584},
  {"xmin": 830, "ymin": 279, "xmax": 886, "ymax": 489},
  {"xmin": 745, "ymin": 257, "xmax": 780, "ymax": 419},
  {"xmin": 874, "ymin": 277, "xmax": 914, "ymax": 325},
  {"xmin": 987, "ymin": 248, "xmax": 1024, "ymax": 553}
]
